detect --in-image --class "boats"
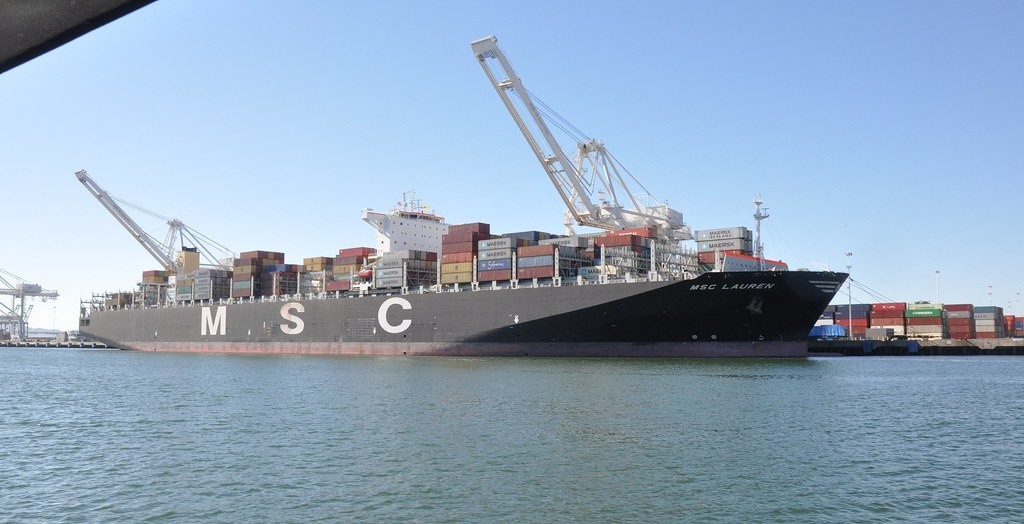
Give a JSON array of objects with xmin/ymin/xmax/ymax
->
[{"xmin": 77, "ymin": 187, "xmax": 849, "ymax": 361}]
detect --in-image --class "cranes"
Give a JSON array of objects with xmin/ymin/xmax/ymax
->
[
  {"xmin": 0, "ymin": 268, "xmax": 60, "ymax": 337},
  {"xmin": 473, "ymin": 36, "xmax": 685, "ymax": 233},
  {"xmin": 75, "ymin": 170, "xmax": 238, "ymax": 278}
]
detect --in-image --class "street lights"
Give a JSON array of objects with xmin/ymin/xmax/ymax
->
[
  {"xmin": 987, "ymin": 284, "xmax": 1021, "ymax": 315},
  {"xmin": 844, "ymin": 252, "xmax": 853, "ymax": 340},
  {"xmin": 934, "ymin": 270, "xmax": 940, "ymax": 305}
]
